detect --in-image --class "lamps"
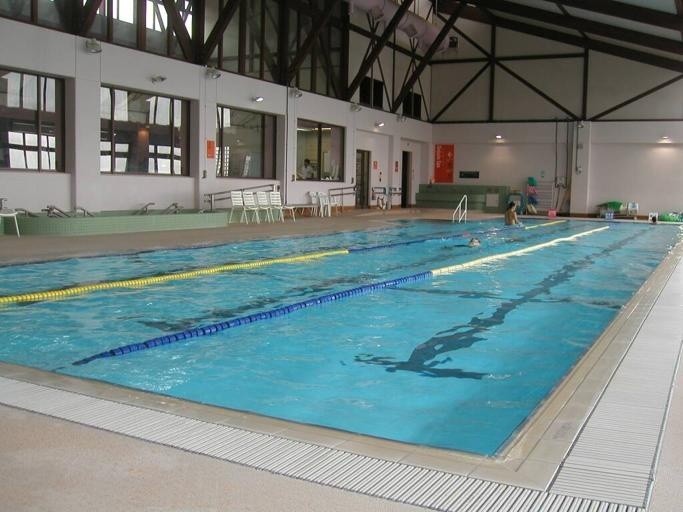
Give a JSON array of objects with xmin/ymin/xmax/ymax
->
[
  {"xmin": 255, "ymin": 96, "xmax": 264, "ymax": 102},
  {"xmin": 495, "ymin": 135, "xmax": 503, "ymax": 139},
  {"xmin": 207, "ymin": 67, "xmax": 221, "ymax": 79},
  {"xmin": 352, "ymin": 102, "xmax": 361, "ymax": 112},
  {"xmin": 375, "ymin": 120, "xmax": 384, "ymax": 128},
  {"xmin": 294, "ymin": 87, "xmax": 302, "ymax": 98},
  {"xmin": 85, "ymin": 37, "xmax": 101, "ymax": 54}
]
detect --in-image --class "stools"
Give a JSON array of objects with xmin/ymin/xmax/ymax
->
[{"xmin": 1, "ymin": 212, "xmax": 21, "ymax": 238}]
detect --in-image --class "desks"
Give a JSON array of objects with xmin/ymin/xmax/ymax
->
[{"xmin": 285, "ymin": 204, "xmax": 318, "ymax": 217}]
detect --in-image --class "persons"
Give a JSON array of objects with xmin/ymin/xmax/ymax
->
[
  {"xmin": 303, "ymin": 158, "xmax": 314, "ymax": 180},
  {"xmin": 505, "ymin": 202, "xmax": 520, "ymax": 225},
  {"xmin": 469, "ymin": 237, "xmax": 481, "ymax": 247}
]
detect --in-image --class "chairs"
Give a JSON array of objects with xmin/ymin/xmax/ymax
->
[
  {"xmin": 309, "ymin": 190, "xmax": 339, "ymax": 218},
  {"xmin": 256, "ymin": 191, "xmax": 275, "ymax": 223},
  {"xmin": 228, "ymin": 190, "xmax": 250, "ymax": 223},
  {"xmin": 243, "ymin": 191, "xmax": 261, "ymax": 224},
  {"xmin": 270, "ymin": 192, "xmax": 296, "ymax": 222}
]
